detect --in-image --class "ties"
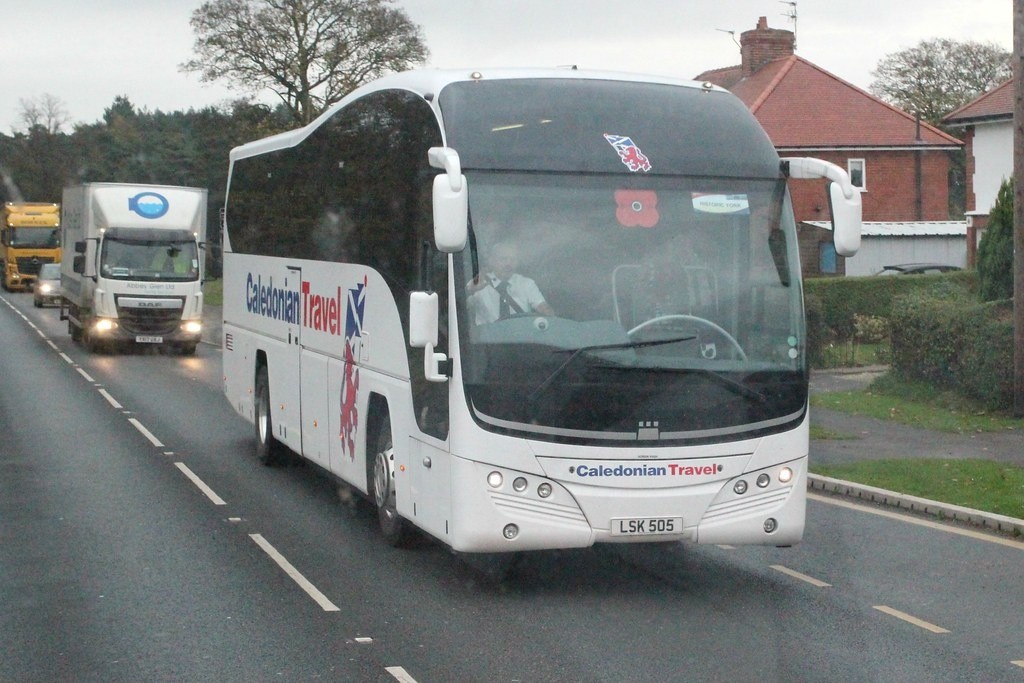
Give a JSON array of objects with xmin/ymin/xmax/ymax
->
[{"xmin": 499, "ymin": 283, "xmax": 510, "ymax": 319}]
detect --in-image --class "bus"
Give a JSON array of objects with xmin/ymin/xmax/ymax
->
[{"xmin": 221, "ymin": 67, "xmax": 862, "ymax": 558}]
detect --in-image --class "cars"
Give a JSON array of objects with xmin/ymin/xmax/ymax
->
[
  {"xmin": 33, "ymin": 263, "xmax": 62, "ymax": 307},
  {"xmin": 874, "ymin": 262, "xmax": 963, "ymax": 274}
]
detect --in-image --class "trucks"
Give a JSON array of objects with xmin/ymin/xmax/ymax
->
[
  {"xmin": 0, "ymin": 200, "xmax": 62, "ymax": 292},
  {"xmin": 59, "ymin": 181, "xmax": 208, "ymax": 356}
]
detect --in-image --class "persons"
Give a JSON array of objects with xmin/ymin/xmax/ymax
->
[
  {"xmin": 464, "ymin": 241, "xmax": 553, "ymax": 327},
  {"xmin": 111, "ymin": 249, "xmax": 132, "ymax": 268}
]
